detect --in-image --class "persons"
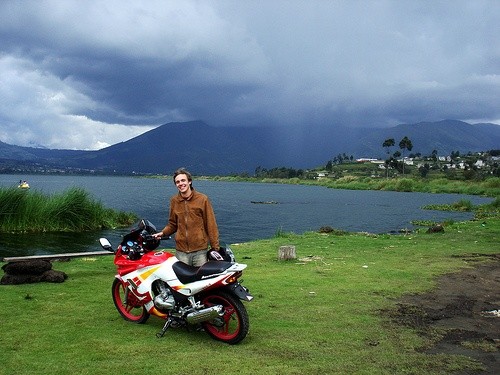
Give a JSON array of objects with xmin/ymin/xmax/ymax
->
[{"xmin": 152, "ymin": 168, "xmax": 220, "ymax": 333}]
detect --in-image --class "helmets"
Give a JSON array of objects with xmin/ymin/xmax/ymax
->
[{"xmin": 207, "ymin": 238, "xmax": 236, "ymax": 263}]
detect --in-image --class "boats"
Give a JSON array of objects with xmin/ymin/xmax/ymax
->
[{"xmin": 17, "ymin": 183, "xmax": 30, "ymax": 189}]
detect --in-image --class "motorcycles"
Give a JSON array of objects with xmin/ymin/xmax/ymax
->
[{"xmin": 99, "ymin": 218, "xmax": 254, "ymax": 345}]
